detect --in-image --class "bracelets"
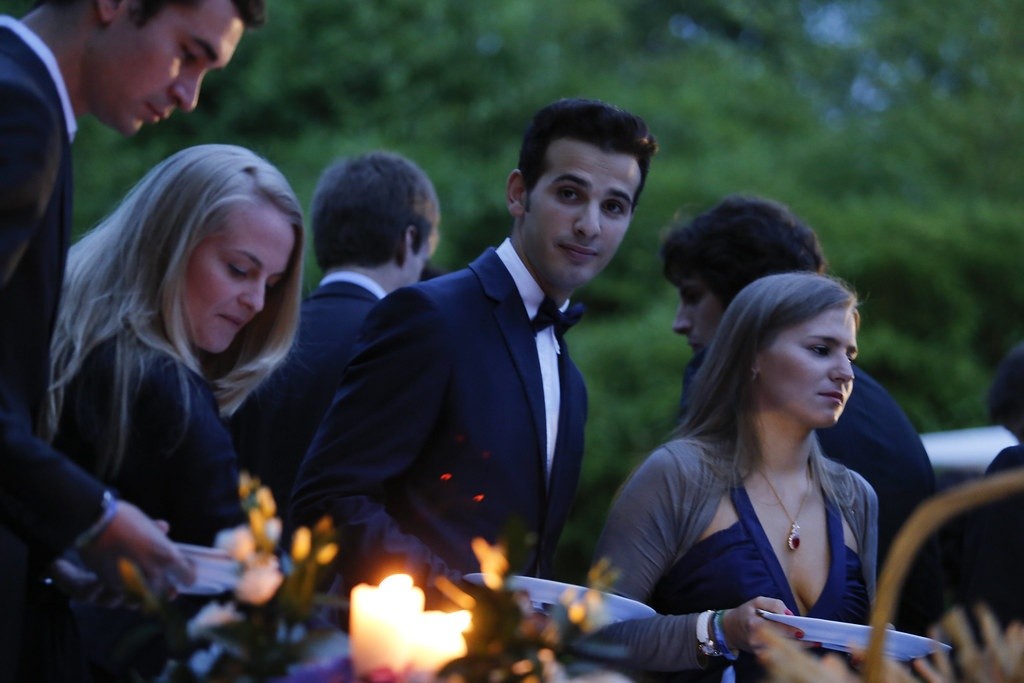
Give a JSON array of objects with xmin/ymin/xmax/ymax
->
[
  {"xmin": 712, "ymin": 609, "xmax": 739, "ymax": 662},
  {"xmin": 69, "ymin": 490, "xmax": 115, "ymax": 552}
]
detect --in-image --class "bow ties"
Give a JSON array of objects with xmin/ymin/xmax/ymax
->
[{"xmin": 528, "ymin": 294, "xmax": 585, "ymax": 342}]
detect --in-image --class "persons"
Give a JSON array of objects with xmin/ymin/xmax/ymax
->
[
  {"xmin": 228, "ymin": 147, "xmax": 443, "ymax": 510},
  {"xmin": 945, "ymin": 345, "xmax": 1024, "ymax": 649},
  {"xmin": 292, "ymin": 96, "xmax": 656, "ymax": 683},
  {"xmin": 655, "ymin": 184, "xmax": 948, "ymax": 637},
  {"xmin": 41, "ymin": 146, "xmax": 349, "ymax": 683},
  {"xmin": 0, "ymin": 0, "xmax": 270, "ymax": 683},
  {"xmin": 583, "ymin": 270, "xmax": 898, "ymax": 683}
]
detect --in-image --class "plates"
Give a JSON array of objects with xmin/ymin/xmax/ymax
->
[
  {"xmin": 755, "ymin": 608, "xmax": 953, "ymax": 663},
  {"xmin": 166, "ymin": 541, "xmax": 245, "ymax": 597},
  {"xmin": 461, "ymin": 572, "xmax": 657, "ymax": 636}
]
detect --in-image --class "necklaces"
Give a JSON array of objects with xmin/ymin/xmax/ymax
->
[{"xmin": 753, "ymin": 461, "xmax": 813, "ymax": 551}]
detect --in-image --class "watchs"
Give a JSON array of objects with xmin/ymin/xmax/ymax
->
[{"xmin": 696, "ymin": 611, "xmax": 722, "ymax": 659}]
